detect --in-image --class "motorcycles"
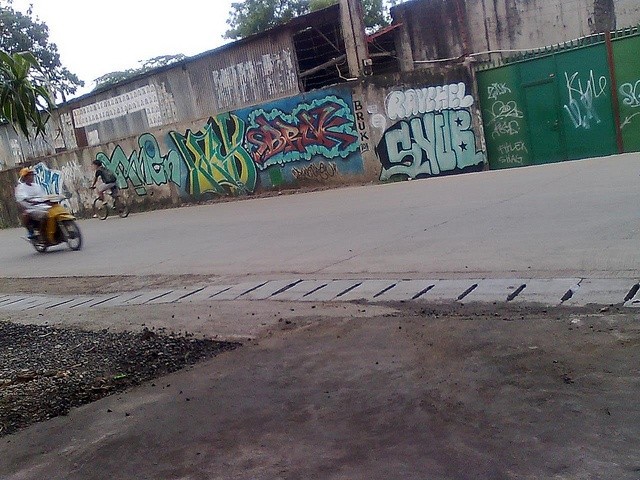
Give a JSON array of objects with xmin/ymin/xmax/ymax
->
[{"xmin": 22, "ymin": 191, "xmax": 82, "ymax": 253}]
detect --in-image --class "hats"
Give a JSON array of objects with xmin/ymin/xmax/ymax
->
[{"xmin": 18, "ymin": 167, "xmax": 33, "ymax": 177}]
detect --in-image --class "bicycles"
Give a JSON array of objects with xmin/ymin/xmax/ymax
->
[{"xmin": 89, "ymin": 186, "xmax": 130, "ymax": 220}]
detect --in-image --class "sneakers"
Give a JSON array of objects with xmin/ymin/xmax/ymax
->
[
  {"xmin": 98, "ymin": 201, "xmax": 107, "ymax": 211},
  {"xmin": 28, "ymin": 230, "xmax": 34, "ymax": 239}
]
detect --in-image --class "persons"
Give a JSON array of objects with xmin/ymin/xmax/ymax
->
[
  {"xmin": 14, "ymin": 168, "xmax": 57, "ymax": 244},
  {"xmin": 91, "ymin": 160, "xmax": 127, "ymax": 217},
  {"xmin": 18, "ymin": 205, "xmax": 35, "ymax": 239}
]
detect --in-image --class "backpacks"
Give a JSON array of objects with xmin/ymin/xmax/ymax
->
[{"xmin": 98, "ymin": 167, "xmax": 116, "ymax": 183}]
